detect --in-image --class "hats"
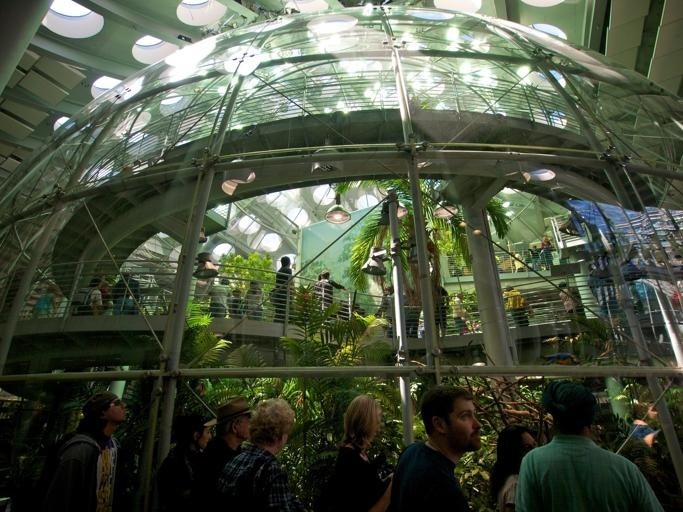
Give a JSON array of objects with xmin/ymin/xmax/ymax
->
[
  {"xmin": 204, "ymin": 393, "xmax": 254, "ymax": 428},
  {"xmin": 83, "ymin": 391, "xmax": 118, "ymax": 412}
]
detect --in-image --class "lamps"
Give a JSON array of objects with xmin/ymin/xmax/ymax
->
[
  {"xmin": 323, "ymin": 192, "xmax": 459, "ymax": 225},
  {"xmin": 192, "ymin": 253, "xmax": 218, "ymax": 279}
]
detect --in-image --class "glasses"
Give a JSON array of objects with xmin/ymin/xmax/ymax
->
[{"xmin": 110, "ymin": 398, "xmax": 122, "ymax": 408}]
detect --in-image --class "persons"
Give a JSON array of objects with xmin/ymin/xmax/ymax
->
[
  {"xmin": 217, "ymin": 397, "xmax": 295, "ymax": 511},
  {"xmin": 500, "ymin": 286, "xmax": 533, "ymax": 327},
  {"xmin": 374, "ymin": 277, "xmax": 481, "ymax": 337},
  {"xmin": 149, "ymin": 395, "xmax": 252, "ymax": 511},
  {"xmin": 488, "ymin": 380, "xmax": 669, "ymax": 511},
  {"xmin": 83, "ymin": 272, "xmax": 140, "ymax": 316},
  {"xmin": 55, "ymin": 391, "xmax": 127, "ymax": 512},
  {"xmin": 203, "ymin": 279, "xmax": 265, "ymax": 319},
  {"xmin": 527, "ymin": 234, "xmax": 556, "ymax": 272},
  {"xmin": 387, "ymin": 385, "xmax": 483, "ymax": 511},
  {"xmin": 558, "ymin": 281, "xmax": 578, "ymax": 319},
  {"xmin": 32, "ymin": 284, "xmax": 58, "ymax": 319},
  {"xmin": 274, "ymin": 256, "xmax": 346, "ymax": 324},
  {"xmin": 327, "ymin": 395, "xmax": 393, "ymax": 511}
]
